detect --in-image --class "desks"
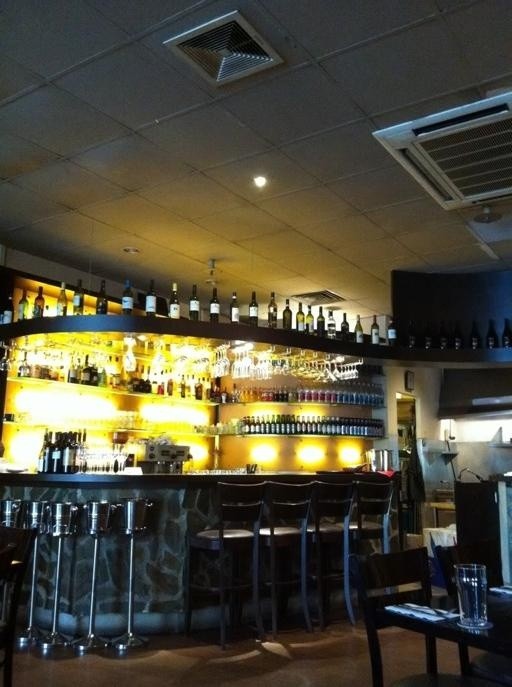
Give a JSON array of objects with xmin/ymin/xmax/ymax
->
[{"xmin": 362, "ymin": 583, "xmax": 512, "ymax": 687}]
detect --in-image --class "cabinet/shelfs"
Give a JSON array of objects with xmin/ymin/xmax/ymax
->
[{"xmin": 0, "ymin": 313, "xmax": 512, "ymax": 476}]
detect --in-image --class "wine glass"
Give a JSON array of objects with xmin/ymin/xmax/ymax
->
[
  {"xmin": 74, "ymin": 448, "xmax": 129, "ymax": 474},
  {"xmin": 275, "ymin": 348, "xmax": 359, "ymax": 381},
  {"xmin": 18, "ymin": 347, "xmax": 32, "ymax": 376},
  {"xmin": 123, "ymin": 337, "xmax": 137, "ymax": 371},
  {"xmin": 172, "ymin": 344, "xmax": 273, "ymax": 380},
  {"xmin": 0, "ymin": 345, "xmax": 11, "ymax": 372}
]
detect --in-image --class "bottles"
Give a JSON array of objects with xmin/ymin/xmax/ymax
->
[
  {"xmin": 266, "ymin": 415, "xmax": 271, "ymax": 434},
  {"xmin": 291, "ymin": 415, "xmax": 296, "ymax": 435},
  {"xmin": 367, "ymin": 382, "xmax": 371, "ymax": 407},
  {"xmin": 73, "ymin": 279, "xmax": 83, "ymax": 316},
  {"xmin": 371, "ymin": 383, "xmax": 375, "ymax": 407},
  {"xmin": 331, "ymin": 416, "xmax": 336, "ymax": 435},
  {"xmin": 357, "ymin": 382, "xmax": 362, "ymax": 406},
  {"xmin": 271, "ymin": 415, "xmax": 276, "ymax": 434},
  {"xmin": 209, "ymin": 287, "xmax": 220, "ymax": 322},
  {"xmin": 36, "ymin": 428, "xmax": 88, "ymax": 474},
  {"xmin": 249, "ymin": 291, "xmax": 258, "ymax": 327},
  {"xmin": 347, "ymin": 381, "xmax": 352, "ymax": 405},
  {"xmin": 96, "ymin": 280, "xmax": 108, "ymax": 315},
  {"xmin": 56, "ymin": 281, "xmax": 67, "ymax": 316},
  {"xmin": 273, "ymin": 384, "xmax": 278, "ymax": 402},
  {"xmin": 317, "ymin": 416, "xmax": 322, "ymax": 434},
  {"xmin": 168, "ymin": 282, "xmax": 180, "ymax": 319},
  {"xmin": 32, "ymin": 287, "xmax": 44, "ymax": 318},
  {"xmin": 317, "ymin": 307, "xmax": 325, "ymax": 338},
  {"xmin": 406, "ymin": 320, "xmax": 418, "ymax": 352},
  {"xmin": 302, "ymin": 416, "xmax": 307, "ymax": 434},
  {"xmin": 145, "ymin": 279, "xmax": 156, "ymax": 316},
  {"xmin": 286, "ymin": 415, "xmax": 291, "ymax": 435},
  {"xmin": 238, "ymin": 383, "xmax": 262, "ymax": 403},
  {"xmin": 380, "ymin": 384, "xmax": 385, "ymax": 407},
  {"xmin": 251, "ymin": 417, "xmax": 256, "ymax": 434},
  {"xmin": 293, "ymin": 384, "xmax": 296, "ymax": 402},
  {"xmin": 318, "ymin": 380, "xmax": 325, "ymax": 404},
  {"xmin": 325, "ymin": 381, "xmax": 331, "ymax": 404},
  {"xmin": 342, "ymin": 380, "xmax": 347, "ymax": 405},
  {"xmin": 3, "ymin": 291, "xmax": 14, "ymax": 324},
  {"xmin": 260, "ymin": 387, "xmax": 274, "ymax": 402},
  {"xmin": 278, "ymin": 384, "xmax": 283, "ymax": 402},
  {"xmin": 312, "ymin": 416, "xmax": 317, "ymax": 434},
  {"xmin": 452, "ymin": 320, "xmax": 464, "ymax": 352},
  {"xmin": 230, "ymin": 383, "xmax": 238, "ymax": 403},
  {"xmin": 283, "ymin": 298, "xmax": 292, "ymax": 331},
  {"xmin": 281, "ymin": 415, "xmax": 286, "ymax": 435},
  {"xmin": 350, "ymin": 417, "xmax": 353, "ymax": 436},
  {"xmin": 288, "ymin": 384, "xmax": 292, "ymax": 402},
  {"xmin": 296, "ymin": 302, "xmax": 305, "ymax": 334},
  {"xmin": 17, "ymin": 288, "xmax": 28, "ymax": 321},
  {"xmin": 268, "ymin": 290, "xmax": 278, "ymax": 329},
  {"xmin": 470, "ymin": 320, "xmax": 482, "ymax": 350},
  {"xmin": 437, "ymin": 320, "xmax": 451, "ymax": 352},
  {"xmin": 341, "ymin": 313, "xmax": 350, "ymax": 342},
  {"xmin": 283, "ymin": 384, "xmax": 288, "ymax": 402},
  {"xmin": 17, "ymin": 350, "xmax": 211, "ymax": 400},
  {"xmin": 369, "ymin": 418, "xmax": 385, "ymax": 437},
  {"xmin": 341, "ymin": 416, "xmax": 346, "ymax": 436},
  {"xmin": 326, "ymin": 416, "xmax": 331, "ymax": 435},
  {"xmin": 357, "ymin": 418, "xmax": 361, "ymax": 436},
  {"xmin": 230, "ymin": 291, "xmax": 240, "ymax": 325},
  {"xmin": 371, "ymin": 315, "xmax": 380, "ymax": 345},
  {"xmin": 502, "ymin": 317, "xmax": 511, "ymax": 347},
  {"xmin": 328, "ymin": 309, "xmax": 336, "ymax": 340},
  {"xmin": 345, "ymin": 417, "xmax": 350, "ymax": 436},
  {"xmin": 354, "ymin": 314, "xmax": 364, "ymax": 344},
  {"xmin": 487, "ymin": 318, "xmax": 499, "ymax": 351},
  {"xmin": 303, "ymin": 378, "xmax": 311, "ymax": 403},
  {"xmin": 388, "ymin": 318, "xmax": 400, "ymax": 347},
  {"xmin": 336, "ymin": 416, "xmax": 341, "ymax": 435},
  {"xmin": 362, "ymin": 382, "xmax": 367, "ymax": 406},
  {"xmin": 336, "ymin": 380, "xmax": 342, "ymax": 404},
  {"xmin": 296, "ymin": 377, "xmax": 303, "ymax": 402},
  {"xmin": 220, "ymin": 387, "xmax": 230, "ymax": 403},
  {"xmin": 331, "ymin": 380, "xmax": 336, "ymax": 404},
  {"xmin": 375, "ymin": 383, "xmax": 380, "ymax": 407},
  {"xmin": 256, "ymin": 417, "xmax": 261, "ymax": 434},
  {"xmin": 352, "ymin": 381, "xmax": 357, "ymax": 405},
  {"xmin": 188, "ymin": 284, "xmax": 199, "ymax": 321},
  {"xmin": 306, "ymin": 305, "xmax": 315, "ymax": 336},
  {"xmin": 122, "ymin": 280, "xmax": 133, "ymax": 315},
  {"xmin": 353, "ymin": 418, "xmax": 357, "ymax": 436},
  {"xmin": 365, "ymin": 418, "xmax": 369, "ymax": 436},
  {"xmin": 422, "ymin": 319, "xmax": 434, "ymax": 353},
  {"xmin": 307, "ymin": 416, "xmax": 312, "ymax": 434},
  {"xmin": 296, "ymin": 416, "xmax": 302, "ymax": 434},
  {"xmin": 246, "ymin": 416, "xmax": 251, "ymax": 434},
  {"xmin": 242, "ymin": 417, "xmax": 246, "ymax": 435},
  {"xmin": 311, "ymin": 379, "xmax": 318, "ymax": 403},
  {"xmin": 276, "ymin": 415, "xmax": 281, "ymax": 435},
  {"xmin": 361, "ymin": 418, "xmax": 365, "ymax": 436},
  {"xmin": 261, "ymin": 416, "xmax": 266, "ymax": 434},
  {"xmin": 321, "ymin": 416, "xmax": 326, "ymax": 435}
]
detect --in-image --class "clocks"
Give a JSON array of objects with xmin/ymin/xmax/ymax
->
[{"xmin": 405, "ymin": 370, "xmax": 414, "ymax": 389}]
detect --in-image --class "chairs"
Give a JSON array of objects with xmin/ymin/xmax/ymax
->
[
  {"xmin": 0, "ymin": 524, "xmax": 39, "ymax": 687},
  {"xmin": 434, "ymin": 546, "xmax": 485, "ymax": 687},
  {"xmin": 357, "ymin": 546, "xmax": 439, "ymax": 687},
  {"xmin": 307, "ymin": 479, "xmax": 358, "ymax": 633},
  {"xmin": 184, "ymin": 481, "xmax": 269, "ymax": 651},
  {"xmin": 260, "ymin": 475, "xmax": 316, "ymax": 640},
  {"xmin": 336, "ymin": 480, "xmax": 399, "ymax": 596}
]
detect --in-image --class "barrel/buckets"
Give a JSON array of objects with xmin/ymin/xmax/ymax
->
[{"xmin": 368, "ymin": 449, "xmax": 392, "ymax": 472}]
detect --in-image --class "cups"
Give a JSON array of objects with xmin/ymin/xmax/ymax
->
[
  {"xmin": 246, "ymin": 464, "xmax": 257, "ymax": 474},
  {"xmin": 453, "ymin": 563, "xmax": 488, "ymax": 627}
]
